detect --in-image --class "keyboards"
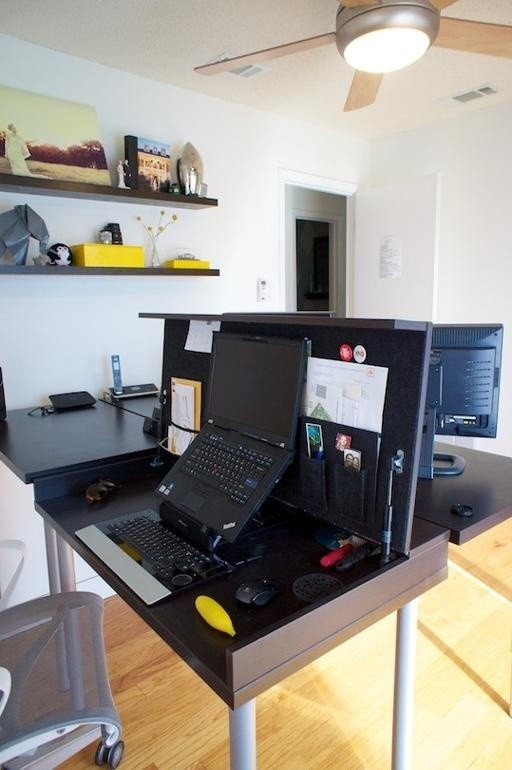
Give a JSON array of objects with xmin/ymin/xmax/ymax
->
[{"xmin": 107, "ymin": 512, "xmax": 225, "ymax": 585}]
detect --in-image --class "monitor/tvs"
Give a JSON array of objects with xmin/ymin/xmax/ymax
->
[{"xmin": 418, "ymin": 324, "xmax": 505, "ymax": 479}]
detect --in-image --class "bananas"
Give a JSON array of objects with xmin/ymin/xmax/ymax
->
[{"xmin": 195, "ymin": 595, "xmax": 237, "ymax": 638}]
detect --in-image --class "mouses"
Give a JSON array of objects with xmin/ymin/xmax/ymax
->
[{"xmin": 235, "ymin": 575, "xmax": 275, "ymax": 606}]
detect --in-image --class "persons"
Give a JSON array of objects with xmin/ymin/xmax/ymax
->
[
  {"xmin": 336, "ymin": 435, "xmax": 347, "ymax": 451},
  {"xmin": 123, "ymin": 158, "xmax": 132, "ymax": 186},
  {"xmin": 353, "ymin": 457, "xmax": 359, "ymax": 471},
  {"xmin": 3, "ymin": 123, "xmax": 53, "ymax": 179},
  {"xmin": 344, "ymin": 453, "xmax": 354, "ymax": 470},
  {"xmin": 117, "ymin": 160, "xmax": 126, "ymax": 187}
]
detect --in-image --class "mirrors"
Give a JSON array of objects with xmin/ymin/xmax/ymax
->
[{"xmin": 313, "ymin": 235, "xmax": 329, "ymax": 293}]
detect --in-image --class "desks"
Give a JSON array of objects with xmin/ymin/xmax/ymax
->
[
  {"xmin": 96, "ymin": 394, "xmax": 162, "ymax": 420},
  {"xmin": 412, "ymin": 438, "xmax": 512, "ymax": 546},
  {"xmin": 31, "ymin": 452, "xmax": 450, "ymax": 770},
  {"xmin": 0, "ymin": 401, "xmax": 158, "ymax": 694}
]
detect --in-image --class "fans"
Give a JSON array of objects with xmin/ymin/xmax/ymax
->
[{"xmin": 193, "ymin": 1, "xmax": 512, "ymax": 112}]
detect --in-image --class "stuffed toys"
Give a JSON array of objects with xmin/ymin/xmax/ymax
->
[{"xmin": 46, "ymin": 243, "xmax": 73, "ymax": 266}]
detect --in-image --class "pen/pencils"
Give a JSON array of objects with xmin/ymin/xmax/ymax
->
[{"xmin": 316, "ymin": 446, "xmax": 324, "ymax": 460}]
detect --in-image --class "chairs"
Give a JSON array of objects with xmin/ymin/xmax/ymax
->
[{"xmin": 1, "ymin": 592, "xmax": 123, "ymax": 769}]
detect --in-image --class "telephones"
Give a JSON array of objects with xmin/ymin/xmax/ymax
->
[{"xmin": 109, "ymin": 355, "xmax": 160, "ymax": 402}]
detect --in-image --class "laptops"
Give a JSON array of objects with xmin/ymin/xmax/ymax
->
[{"xmin": 152, "ymin": 330, "xmax": 308, "ymax": 542}]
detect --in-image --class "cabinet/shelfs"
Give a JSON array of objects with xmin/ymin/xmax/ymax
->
[{"xmin": 0, "ymin": 173, "xmax": 221, "ymax": 277}]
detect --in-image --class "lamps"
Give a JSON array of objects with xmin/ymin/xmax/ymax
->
[{"xmin": 333, "ymin": 1, "xmax": 440, "ymax": 76}]
